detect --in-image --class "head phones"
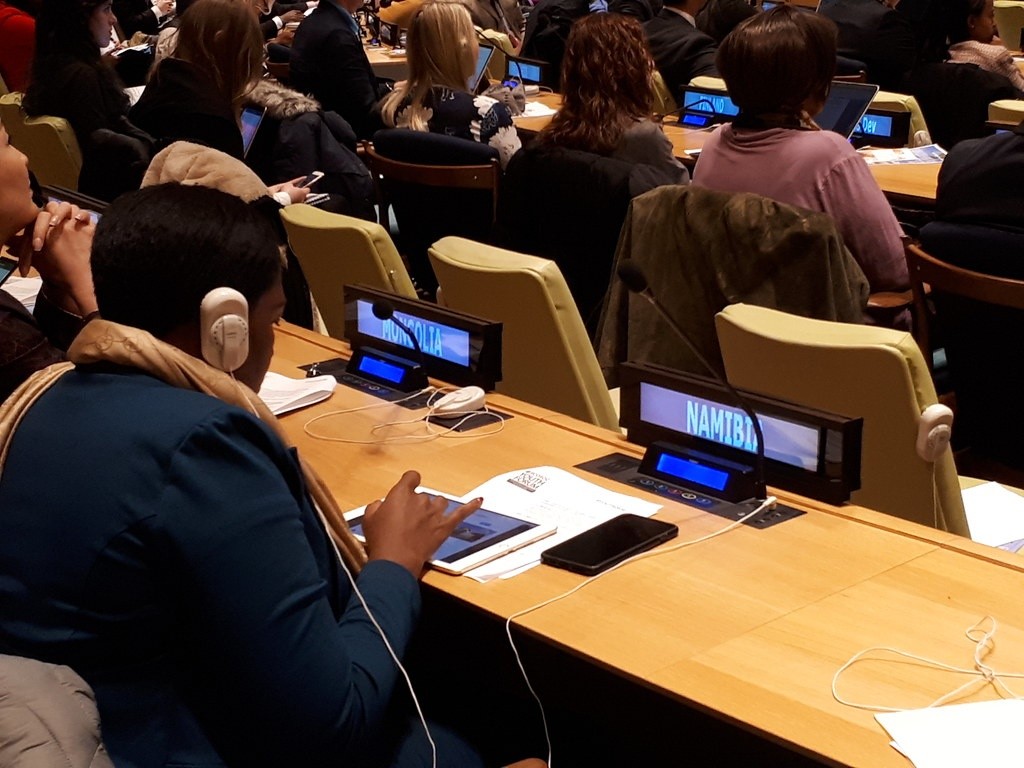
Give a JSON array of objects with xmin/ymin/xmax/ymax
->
[
  {"xmin": 912, "ymin": 131, "xmax": 931, "ymax": 148},
  {"xmin": 431, "ymin": 386, "xmax": 486, "ymax": 418},
  {"xmin": 200, "ymin": 286, "xmax": 250, "ymax": 373},
  {"xmin": 915, "ymin": 404, "xmax": 954, "ymax": 461}
]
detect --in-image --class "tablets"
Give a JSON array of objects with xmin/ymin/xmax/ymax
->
[{"xmin": 342, "ymin": 486, "xmax": 557, "ymax": 574}]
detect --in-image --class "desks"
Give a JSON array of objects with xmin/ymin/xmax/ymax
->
[{"xmin": 3, "ymin": 45, "xmax": 1024, "ymax": 768}]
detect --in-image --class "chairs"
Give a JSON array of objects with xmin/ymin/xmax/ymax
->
[{"xmin": 1, "ymin": 30, "xmax": 1024, "ymax": 544}]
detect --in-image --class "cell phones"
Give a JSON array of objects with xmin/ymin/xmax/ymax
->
[
  {"xmin": 541, "ymin": 514, "xmax": 679, "ymax": 576},
  {"xmin": 0, "ymin": 256, "xmax": 19, "ymax": 287},
  {"xmin": 296, "ymin": 171, "xmax": 324, "ymax": 189}
]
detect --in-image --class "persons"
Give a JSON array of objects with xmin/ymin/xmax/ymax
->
[
  {"xmin": 696, "ymin": 0, "xmax": 759, "ymax": 43},
  {"xmin": 930, "ymin": 119, "xmax": 1024, "ymax": 490},
  {"xmin": 113, "ymin": 0, "xmax": 194, "ymax": 40},
  {"xmin": 815, "ymin": 0, "xmax": 897, "ymax": 55},
  {"xmin": 499, "ymin": 11, "xmax": 689, "ymax": 343},
  {"xmin": 15, "ymin": 0, "xmax": 139, "ymax": 161},
  {"xmin": 943, "ymin": 0, "xmax": 1024, "ymax": 94},
  {"xmin": 370, "ymin": 3, "xmax": 523, "ymax": 173},
  {"xmin": 0, "ymin": 118, "xmax": 102, "ymax": 412},
  {"xmin": 254, "ymin": 0, "xmax": 307, "ymax": 41},
  {"xmin": 349, "ymin": 0, "xmax": 407, "ymax": 49},
  {"xmin": 693, "ymin": 4, "xmax": 911, "ymax": 324},
  {"xmin": 0, "ymin": 179, "xmax": 548, "ymax": 768},
  {"xmin": 126, "ymin": 0, "xmax": 310, "ymax": 204},
  {"xmin": 640, "ymin": 0, "xmax": 722, "ymax": 109},
  {"xmin": 289, "ymin": 0, "xmax": 411, "ymax": 141},
  {"xmin": 463, "ymin": 0, "xmax": 526, "ymax": 48}
]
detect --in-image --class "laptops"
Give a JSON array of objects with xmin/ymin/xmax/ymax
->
[
  {"xmin": 238, "ymin": 104, "xmax": 268, "ymax": 159},
  {"xmin": 761, "ymin": 0, "xmax": 785, "ymax": 12},
  {"xmin": 812, "ymin": 80, "xmax": 878, "ymax": 142},
  {"xmin": 470, "ymin": 43, "xmax": 496, "ymax": 94}
]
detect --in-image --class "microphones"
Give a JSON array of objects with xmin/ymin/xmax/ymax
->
[
  {"xmin": 371, "ymin": 299, "xmax": 424, "ymax": 364},
  {"xmin": 615, "ymin": 257, "xmax": 767, "ymax": 500},
  {"xmin": 360, "ymin": 8, "xmax": 402, "ymax": 49},
  {"xmin": 650, "ymin": 99, "xmax": 716, "ymax": 123}
]
